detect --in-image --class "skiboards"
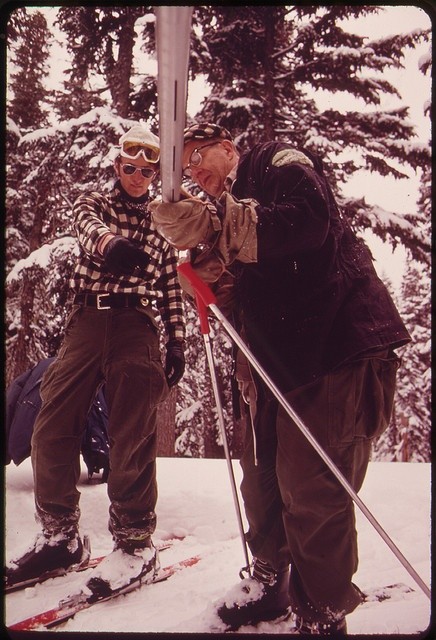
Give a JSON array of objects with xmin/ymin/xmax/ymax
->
[
  {"xmin": 3, "ymin": 536, "xmax": 199, "ymax": 631},
  {"xmin": 356, "ymin": 581, "xmax": 414, "ymax": 602}
]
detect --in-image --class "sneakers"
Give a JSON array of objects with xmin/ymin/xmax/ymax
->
[
  {"xmin": 6, "ymin": 526, "xmax": 83, "ymax": 583},
  {"xmin": 289, "ymin": 617, "xmax": 347, "ymax": 635},
  {"xmin": 217, "ymin": 558, "xmax": 291, "ymax": 627},
  {"xmin": 81, "ymin": 538, "xmax": 156, "ymax": 604}
]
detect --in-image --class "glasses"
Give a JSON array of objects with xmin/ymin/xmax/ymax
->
[
  {"xmin": 182, "ymin": 141, "xmax": 221, "ymax": 181},
  {"xmin": 118, "ymin": 162, "xmax": 157, "ymax": 179},
  {"xmin": 120, "ymin": 138, "xmax": 160, "ymax": 163}
]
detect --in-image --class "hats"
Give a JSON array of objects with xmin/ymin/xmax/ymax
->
[
  {"xmin": 119, "ymin": 126, "xmax": 160, "ymax": 150},
  {"xmin": 184, "ymin": 123, "xmax": 234, "ymax": 143}
]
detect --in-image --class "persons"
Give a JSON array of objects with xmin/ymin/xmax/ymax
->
[
  {"xmin": 150, "ymin": 121, "xmax": 410, "ymax": 634},
  {"xmin": 5, "ymin": 123, "xmax": 186, "ymax": 597}
]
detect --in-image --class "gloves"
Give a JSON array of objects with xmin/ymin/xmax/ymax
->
[
  {"xmin": 177, "ymin": 251, "xmax": 225, "ymax": 298},
  {"xmin": 149, "ymin": 187, "xmax": 222, "ymax": 251},
  {"xmin": 164, "ymin": 340, "xmax": 186, "ymax": 388},
  {"xmin": 103, "ymin": 235, "xmax": 142, "ymax": 276}
]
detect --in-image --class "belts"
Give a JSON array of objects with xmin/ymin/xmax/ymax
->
[{"xmin": 74, "ymin": 293, "xmax": 139, "ymax": 310}]
